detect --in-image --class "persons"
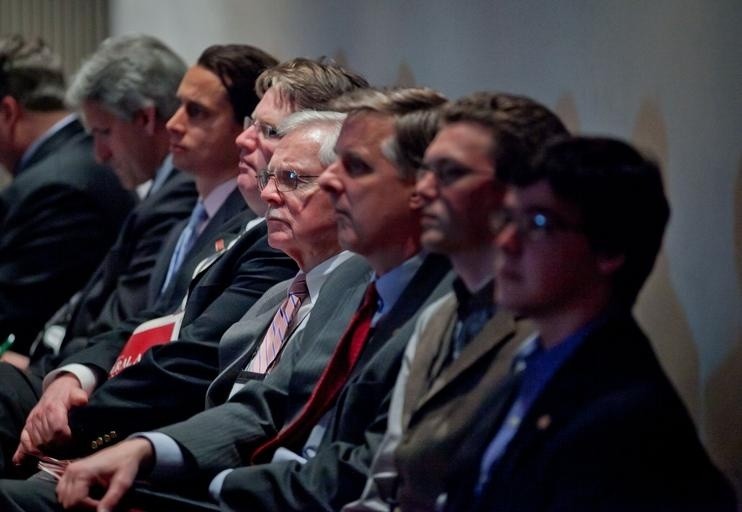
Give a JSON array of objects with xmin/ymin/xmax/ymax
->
[{"xmin": 1, "ymin": 31, "xmax": 742, "ymax": 511}]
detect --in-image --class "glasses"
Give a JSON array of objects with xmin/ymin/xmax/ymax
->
[
  {"xmin": 486, "ymin": 203, "xmax": 581, "ymax": 242},
  {"xmin": 407, "ymin": 154, "xmax": 492, "ymax": 182},
  {"xmin": 242, "ymin": 116, "xmax": 280, "ymax": 141},
  {"xmin": 255, "ymin": 168, "xmax": 319, "ymax": 193}
]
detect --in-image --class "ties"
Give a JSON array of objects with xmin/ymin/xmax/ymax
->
[
  {"xmin": 447, "ymin": 359, "xmax": 527, "ymax": 501},
  {"xmin": 252, "ymin": 280, "xmax": 378, "ymax": 465},
  {"xmin": 161, "ymin": 199, "xmax": 207, "ymax": 295},
  {"xmin": 227, "ymin": 269, "xmax": 308, "ymax": 400}
]
